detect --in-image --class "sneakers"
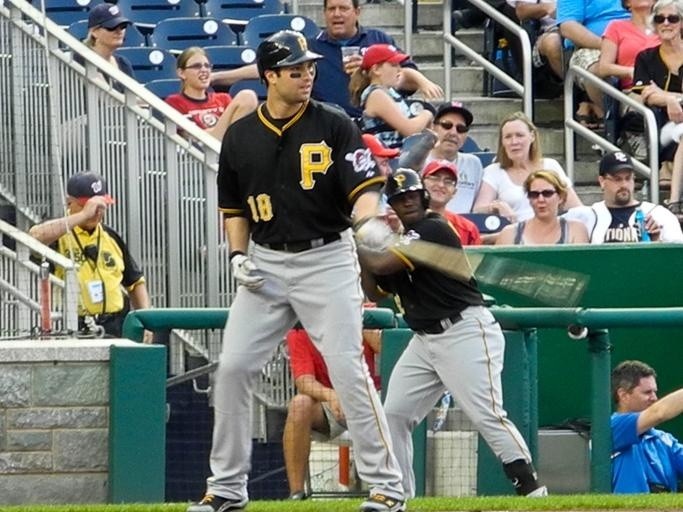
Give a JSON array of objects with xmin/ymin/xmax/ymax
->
[
  {"xmin": 625, "ymin": 130, "xmax": 649, "ymax": 161},
  {"xmin": 185, "ymin": 493, "xmax": 250, "ymax": 512},
  {"xmin": 358, "ymin": 492, "xmax": 409, "ymax": 511},
  {"xmin": 288, "ymin": 490, "xmax": 314, "ymax": 503},
  {"xmin": 526, "ymin": 484, "xmax": 549, "ymax": 497}
]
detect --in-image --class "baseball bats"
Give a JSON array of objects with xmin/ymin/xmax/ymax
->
[
  {"xmin": 379, "ymin": 128, "xmax": 438, "ymax": 198},
  {"xmin": 389, "ymin": 235, "xmax": 589, "ymax": 309}
]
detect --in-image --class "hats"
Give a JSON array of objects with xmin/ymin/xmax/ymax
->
[
  {"xmin": 361, "ymin": 43, "xmax": 411, "ymax": 70},
  {"xmin": 434, "ymin": 99, "xmax": 474, "ymax": 128},
  {"xmin": 600, "ymin": 150, "xmax": 635, "ymax": 177},
  {"xmin": 424, "ymin": 159, "xmax": 459, "ymax": 187},
  {"xmin": 87, "ymin": 2, "xmax": 132, "ymax": 32},
  {"xmin": 362, "ymin": 133, "xmax": 402, "ymax": 159},
  {"xmin": 65, "ymin": 169, "xmax": 117, "ymax": 209}
]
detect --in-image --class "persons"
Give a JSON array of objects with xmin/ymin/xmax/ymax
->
[
  {"xmin": 166, "ymin": 45, "xmax": 258, "ymax": 142},
  {"xmin": 356, "ymin": 168, "xmax": 550, "ymax": 497},
  {"xmin": 187, "ymin": 27, "xmax": 411, "ymax": 509},
  {"xmin": 69, "ymin": 4, "xmax": 136, "ymax": 96},
  {"xmin": 278, "ymin": 321, "xmax": 382, "ymax": 500},
  {"xmin": 26, "ymin": 171, "xmax": 157, "ymax": 345},
  {"xmin": 590, "ymin": 357, "xmax": 682, "ymax": 496},
  {"xmin": 203, "ymin": 0, "xmax": 416, "ymax": 118}
]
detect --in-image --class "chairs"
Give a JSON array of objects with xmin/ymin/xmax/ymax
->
[{"xmin": 27, "ymin": 1, "xmax": 681, "ymax": 244}]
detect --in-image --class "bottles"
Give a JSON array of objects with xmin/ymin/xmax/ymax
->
[{"xmin": 634, "ymin": 207, "xmax": 649, "ymax": 240}]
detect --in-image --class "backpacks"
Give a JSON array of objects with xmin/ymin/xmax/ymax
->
[{"xmin": 483, "ymin": 3, "xmax": 543, "ymax": 98}]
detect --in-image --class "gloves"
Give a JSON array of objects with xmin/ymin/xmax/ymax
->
[
  {"xmin": 353, "ymin": 214, "xmax": 393, "ymax": 250},
  {"xmin": 229, "ymin": 249, "xmax": 267, "ymax": 291}
]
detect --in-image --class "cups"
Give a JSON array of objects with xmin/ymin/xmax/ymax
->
[{"xmin": 340, "ymin": 44, "xmax": 360, "ymax": 74}]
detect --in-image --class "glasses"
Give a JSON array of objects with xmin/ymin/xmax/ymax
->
[
  {"xmin": 526, "ymin": 190, "xmax": 558, "ymax": 199},
  {"xmin": 101, "ymin": 23, "xmax": 129, "ymax": 31},
  {"xmin": 424, "ymin": 175, "xmax": 459, "ymax": 187},
  {"xmin": 182, "ymin": 62, "xmax": 216, "ymax": 70},
  {"xmin": 435, "ymin": 119, "xmax": 471, "ymax": 133},
  {"xmin": 653, "ymin": 13, "xmax": 682, "ymax": 24}
]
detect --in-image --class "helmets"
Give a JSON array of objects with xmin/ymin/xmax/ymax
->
[
  {"xmin": 256, "ymin": 30, "xmax": 324, "ymax": 82},
  {"xmin": 384, "ymin": 168, "xmax": 431, "ymax": 211}
]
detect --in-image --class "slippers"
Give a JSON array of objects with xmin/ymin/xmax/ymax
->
[
  {"xmin": 663, "ymin": 197, "xmax": 682, "ymax": 225},
  {"xmin": 657, "ymin": 167, "xmax": 673, "ymax": 190},
  {"xmin": 575, "ymin": 102, "xmax": 606, "ymax": 130}
]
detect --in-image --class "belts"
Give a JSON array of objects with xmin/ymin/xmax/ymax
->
[
  {"xmin": 79, "ymin": 310, "xmax": 126, "ymax": 325},
  {"xmin": 249, "ymin": 227, "xmax": 350, "ymax": 253},
  {"xmin": 415, "ymin": 312, "xmax": 466, "ymax": 336}
]
